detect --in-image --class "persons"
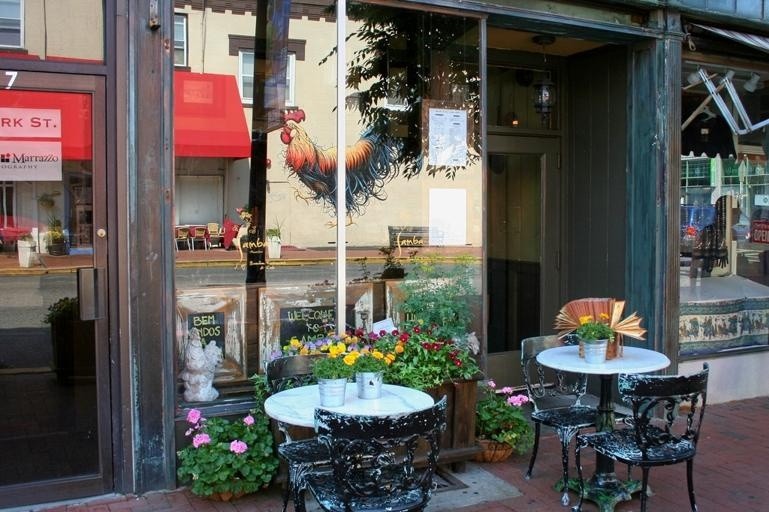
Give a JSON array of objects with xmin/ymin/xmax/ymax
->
[{"xmin": 686, "ymin": 194, "xmax": 745, "ymax": 302}]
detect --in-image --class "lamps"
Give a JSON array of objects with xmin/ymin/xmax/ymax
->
[
  {"xmin": 683, "ymin": 60, "xmax": 764, "ymax": 94},
  {"xmin": 532, "ymin": 35, "xmax": 556, "ymax": 120}
]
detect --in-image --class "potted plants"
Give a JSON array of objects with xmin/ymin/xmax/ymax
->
[
  {"xmin": 578, "ymin": 322, "xmax": 616, "ymax": 361},
  {"xmin": 264, "ymin": 215, "xmax": 288, "ymax": 260},
  {"xmin": 37, "ymin": 295, "xmax": 95, "ymax": 365}
]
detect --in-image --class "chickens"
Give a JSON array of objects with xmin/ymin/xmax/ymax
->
[{"xmin": 277, "ymin": 108, "xmax": 400, "ymax": 230}]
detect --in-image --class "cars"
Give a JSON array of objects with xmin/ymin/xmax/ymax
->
[{"xmin": 681, "ymin": 200, "xmax": 752, "ymax": 258}]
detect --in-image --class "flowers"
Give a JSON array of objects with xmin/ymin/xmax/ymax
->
[
  {"xmin": 351, "ymin": 348, "xmax": 392, "ymax": 371},
  {"xmin": 310, "ymin": 345, "xmax": 355, "ymax": 379},
  {"xmin": 177, "ymin": 408, "xmax": 280, "ymax": 496},
  {"xmin": 476, "ymin": 380, "xmax": 535, "ymax": 455}
]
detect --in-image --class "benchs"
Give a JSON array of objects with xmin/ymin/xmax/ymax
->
[{"xmin": 388, "ymin": 225, "xmax": 445, "ymax": 249}]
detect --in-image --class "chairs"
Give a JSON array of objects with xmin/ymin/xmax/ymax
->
[
  {"xmin": 267, "ymin": 353, "xmax": 396, "ymax": 512},
  {"xmin": 290, "ymin": 394, "xmax": 448, "ymax": 512},
  {"xmin": 175, "ymin": 222, "xmax": 226, "ymax": 252},
  {"xmin": 520, "ymin": 333, "xmax": 596, "ymax": 480},
  {"xmin": 572, "ymin": 362, "xmax": 709, "ymax": 512}
]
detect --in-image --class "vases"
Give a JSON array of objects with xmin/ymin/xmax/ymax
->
[
  {"xmin": 476, "ymin": 435, "xmax": 514, "ymax": 461},
  {"xmin": 206, "ymin": 476, "xmax": 245, "ymax": 502},
  {"xmin": 356, "ymin": 371, "xmax": 383, "ymax": 399},
  {"xmin": 318, "ymin": 378, "xmax": 347, "ymax": 407}
]
